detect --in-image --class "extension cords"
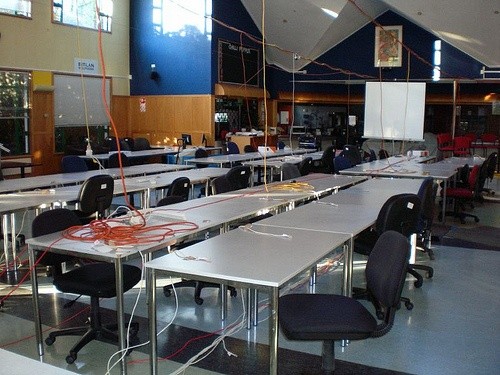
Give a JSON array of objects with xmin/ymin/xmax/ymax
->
[{"xmin": 130, "ymin": 210, "xmax": 142, "ymax": 226}]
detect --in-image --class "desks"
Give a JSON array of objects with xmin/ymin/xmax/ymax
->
[{"xmin": 0, "ymin": 149, "xmax": 487, "ymax": 375}]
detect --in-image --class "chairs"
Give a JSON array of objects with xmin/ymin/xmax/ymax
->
[{"xmin": 31, "ymin": 137, "xmax": 499, "ymax": 375}]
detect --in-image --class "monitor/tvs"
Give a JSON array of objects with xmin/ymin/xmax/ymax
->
[
  {"xmin": 202, "ymin": 134, "xmax": 207, "ymax": 147},
  {"xmin": 182, "ymin": 134, "xmax": 192, "ymax": 149}
]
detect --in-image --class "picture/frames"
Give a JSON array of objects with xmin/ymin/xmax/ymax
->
[{"xmin": 374, "ymin": 26, "xmax": 403, "ymax": 68}]
